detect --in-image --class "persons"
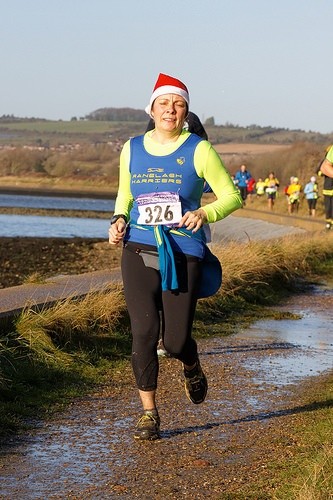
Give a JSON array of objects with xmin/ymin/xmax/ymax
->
[
  {"xmin": 316, "ymin": 144, "xmax": 333, "ymax": 231},
  {"xmin": 108, "ymin": 73, "xmax": 244, "ymax": 439},
  {"xmin": 203, "ymin": 164, "xmax": 319, "ymax": 218}
]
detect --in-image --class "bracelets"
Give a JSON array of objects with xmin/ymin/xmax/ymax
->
[{"xmin": 111, "ymin": 214, "xmax": 128, "ymax": 225}]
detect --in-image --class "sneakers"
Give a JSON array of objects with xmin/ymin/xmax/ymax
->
[
  {"xmin": 134, "ymin": 413, "xmax": 160, "ymax": 440},
  {"xmin": 182, "ymin": 354, "xmax": 208, "ymax": 404}
]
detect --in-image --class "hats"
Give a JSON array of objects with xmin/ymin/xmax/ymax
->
[
  {"xmin": 311, "ymin": 177, "xmax": 316, "ymax": 182},
  {"xmin": 145, "ymin": 73, "xmax": 190, "ymax": 120},
  {"xmin": 293, "ymin": 177, "xmax": 299, "ymax": 182}
]
